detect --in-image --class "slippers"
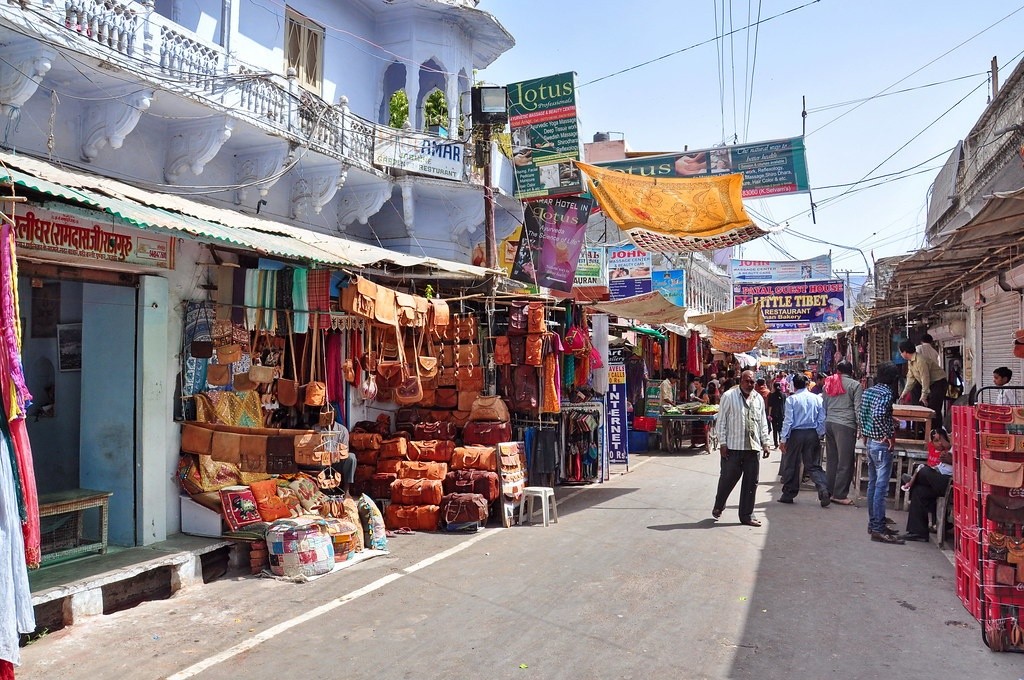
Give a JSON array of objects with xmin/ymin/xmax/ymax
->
[
  {"xmin": 386, "ymin": 531, "xmax": 396, "ymax": 537},
  {"xmin": 831, "ymin": 498, "xmax": 854, "ymax": 505},
  {"xmin": 394, "ymin": 527, "xmax": 415, "ymax": 535}
]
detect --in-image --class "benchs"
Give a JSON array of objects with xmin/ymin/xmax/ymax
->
[{"xmin": 933, "ymin": 477, "xmax": 954, "ymax": 549}]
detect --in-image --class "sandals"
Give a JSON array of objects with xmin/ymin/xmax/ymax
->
[
  {"xmin": 712, "ymin": 507, "xmax": 722, "ymax": 520},
  {"xmin": 748, "ymin": 519, "xmax": 761, "ymax": 526}
]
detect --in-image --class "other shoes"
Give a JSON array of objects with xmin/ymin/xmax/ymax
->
[
  {"xmin": 777, "ymin": 498, "xmax": 792, "ymax": 503},
  {"xmin": 900, "ymin": 533, "xmax": 929, "ymax": 542},
  {"xmin": 821, "ymin": 491, "xmax": 830, "ymax": 507},
  {"xmin": 871, "ymin": 531, "xmax": 904, "ymax": 544},
  {"xmin": 868, "ymin": 527, "xmax": 899, "ymax": 535}
]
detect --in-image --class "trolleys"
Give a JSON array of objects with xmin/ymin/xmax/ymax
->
[{"xmin": 973, "ymin": 387, "xmax": 1024, "ymax": 652}]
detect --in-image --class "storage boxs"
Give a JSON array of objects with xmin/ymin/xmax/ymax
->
[
  {"xmin": 628, "ymin": 416, "xmax": 662, "ymax": 454},
  {"xmin": 950, "ymin": 405, "xmax": 1024, "ymax": 630}
]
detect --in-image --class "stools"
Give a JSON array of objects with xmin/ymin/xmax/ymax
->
[{"xmin": 518, "ymin": 487, "xmax": 558, "ymax": 528}]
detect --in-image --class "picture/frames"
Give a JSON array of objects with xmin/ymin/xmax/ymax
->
[{"xmin": 58, "ymin": 322, "xmax": 81, "ymax": 371}]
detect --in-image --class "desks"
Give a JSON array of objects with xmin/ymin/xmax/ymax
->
[
  {"xmin": 820, "ymin": 403, "xmax": 938, "ymax": 511},
  {"xmin": 37, "ymin": 488, "xmax": 114, "ymax": 561}
]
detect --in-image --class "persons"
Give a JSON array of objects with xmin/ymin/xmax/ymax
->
[
  {"xmin": 609, "ymin": 268, "xmax": 630, "ymax": 279},
  {"xmin": 683, "ymin": 153, "xmax": 731, "ymax": 173},
  {"xmin": 816, "ymin": 298, "xmax": 844, "ymax": 323},
  {"xmin": 654, "ymin": 334, "xmax": 1019, "ymax": 544},
  {"xmin": 312, "ymin": 409, "xmax": 357, "ymax": 498}
]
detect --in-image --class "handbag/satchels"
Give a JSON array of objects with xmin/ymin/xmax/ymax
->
[
  {"xmin": 182, "ymin": 274, "xmax": 605, "ymax": 530},
  {"xmin": 976, "ymin": 404, "xmax": 1024, "ymax": 652}
]
